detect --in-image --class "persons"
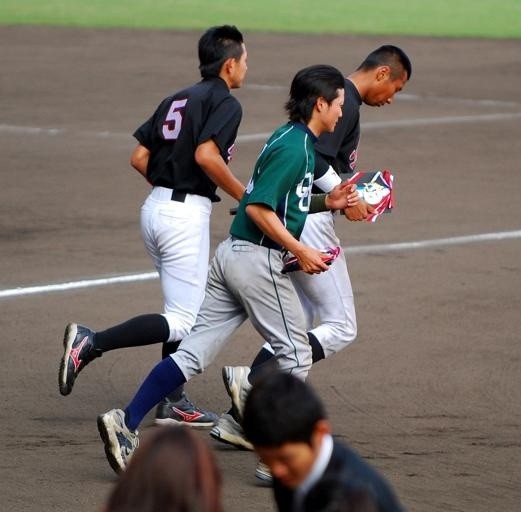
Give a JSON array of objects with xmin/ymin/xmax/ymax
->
[
  {"xmin": 58, "ymin": 25, "xmax": 250, "ymax": 428},
  {"xmin": 101, "ymin": 423, "xmax": 224, "ymax": 511},
  {"xmin": 209, "ymin": 45, "xmax": 413, "ymax": 451},
  {"xmin": 240, "ymin": 368, "xmax": 405, "ymax": 512},
  {"xmin": 96, "ymin": 62, "xmax": 359, "ymax": 484}
]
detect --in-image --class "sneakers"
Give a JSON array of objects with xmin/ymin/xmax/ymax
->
[
  {"xmin": 154, "ymin": 395, "xmax": 218, "ymax": 425},
  {"xmin": 58, "ymin": 322, "xmax": 97, "ymax": 395},
  {"xmin": 209, "ymin": 414, "xmax": 257, "ymax": 449},
  {"xmin": 220, "ymin": 365, "xmax": 254, "ymax": 418},
  {"xmin": 95, "ymin": 408, "xmax": 138, "ymax": 476},
  {"xmin": 254, "ymin": 462, "xmax": 274, "ymax": 483}
]
